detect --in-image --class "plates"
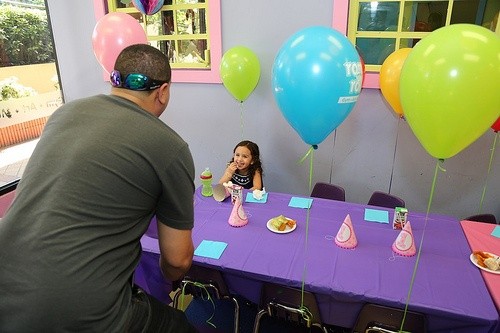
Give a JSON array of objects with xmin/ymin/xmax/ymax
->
[
  {"xmin": 266, "ymin": 218, "xmax": 296, "ymax": 233},
  {"xmin": 470, "ymin": 251, "xmax": 500, "ymax": 274}
]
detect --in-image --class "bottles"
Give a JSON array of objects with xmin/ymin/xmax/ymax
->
[{"xmin": 199, "ymin": 168, "xmax": 213, "ymax": 196}]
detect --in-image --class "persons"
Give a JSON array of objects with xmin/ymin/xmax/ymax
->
[
  {"xmin": 0, "ymin": 44, "xmax": 194, "ymax": 332},
  {"xmin": 219, "ymin": 141, "xmax": 262, "ymax": 190}
]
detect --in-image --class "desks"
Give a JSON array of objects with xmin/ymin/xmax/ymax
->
[{"xmin": 132, "ymin": 183, "xmax": 500, "ymax": 333}]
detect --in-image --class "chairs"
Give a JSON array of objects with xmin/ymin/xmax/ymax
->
[
  {"xmin": 253, "ymin": 284, "xmax": 327, "ymax": 333},
  {"xmin": 351, "ymin": 303, "xmax": 426, "ymax": 333},
  {"xmin": 171, "ymin": 261, "xmax": 239, "ymax": 333},
  {"xmin": 310, "ymin": 183, "xmax": 345, "ymax": 201},
  {"xmin": 367, "ymin": 191, "xmax": 405, "ymax": 209}
]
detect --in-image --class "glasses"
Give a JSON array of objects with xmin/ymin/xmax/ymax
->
[{"xmin": 109, "ymin": 70, "xmax": 169, "ymax": 91}]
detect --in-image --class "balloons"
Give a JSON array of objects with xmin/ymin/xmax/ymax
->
[
  {"xmin": 219, "ymin": 46, "xmax": 260, "ymax": 103},
  {"xmin": 400, "ymin": 24, "xmax": 500, "ymax": 163},
  {"xmin": 92, "ymin": 12, "xmax": 147, "ymax": 75},
  {"xmin": 272, "ymin": 26, "xmax": 363, "ymax": 149},
  {"xmin": 380, "ymin": 48, "xmax": 412, "ymax": 118},
  {"xmin": 132, "ymin": 0, "xmax": 164, "ymax": 15},
  {"xmin": 377, "ymin": 21, "xmax": 430, "ymax": 65}
]
